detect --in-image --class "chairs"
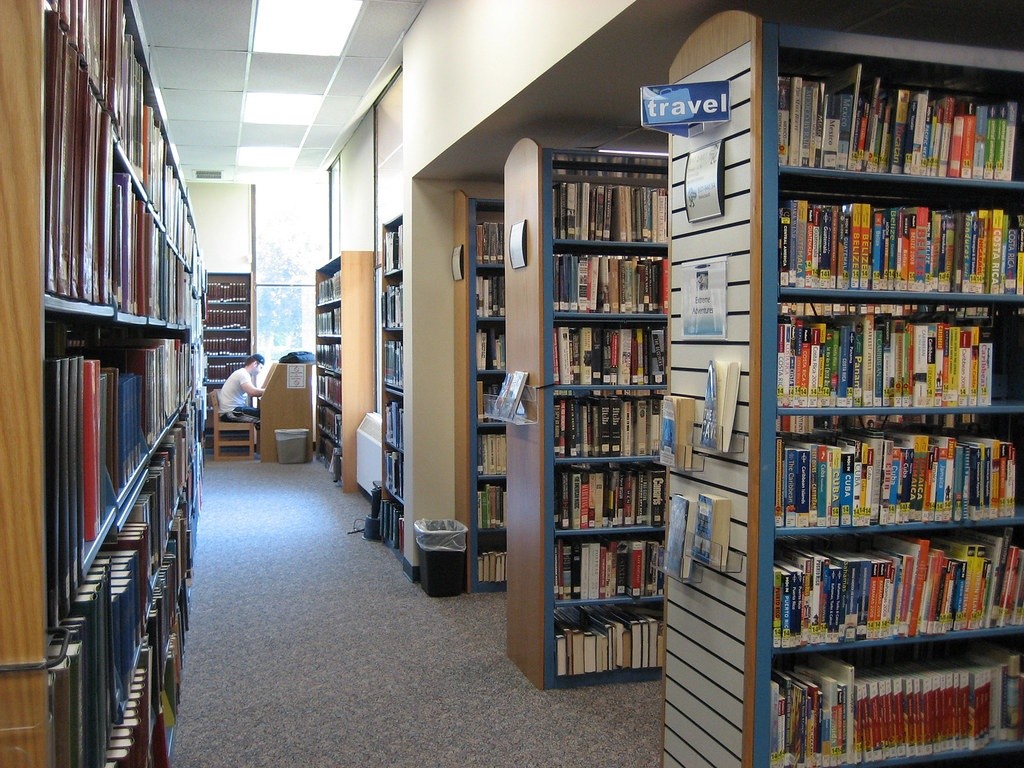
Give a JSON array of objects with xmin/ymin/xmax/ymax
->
[{"xmin": 211, "ymin": 391, "xmax": 255, "ymax": 460}]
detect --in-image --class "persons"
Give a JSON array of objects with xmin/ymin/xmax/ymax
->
[{"xmin": 218, "ymin": 353, "xmax": 265, "ymax": 460}]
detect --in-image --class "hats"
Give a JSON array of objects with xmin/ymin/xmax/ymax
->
[{"xmin": 253, "ymin": 354, "xmax": 265, "ymax": 365}]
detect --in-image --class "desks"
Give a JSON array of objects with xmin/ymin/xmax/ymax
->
[{"xmin": 259, "ymin": 359, "xmax": 316, "ymax": 464}]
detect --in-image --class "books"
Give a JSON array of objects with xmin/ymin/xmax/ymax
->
[{"xmin": 48, "ymin": 0, "xmax": 1024, "ymax": 768}]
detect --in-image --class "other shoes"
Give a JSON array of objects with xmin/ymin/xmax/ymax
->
[{"xmin": 255, "ymin": 452, "xmax": 261, "ymax": 460}]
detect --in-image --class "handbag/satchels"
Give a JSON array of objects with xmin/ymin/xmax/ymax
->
[{"xmin": 279, "ymin": 351, "xmax": 315, "ymax": 364}]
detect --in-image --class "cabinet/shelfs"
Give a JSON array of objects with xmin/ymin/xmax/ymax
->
[
  {"xmin": 382, "ymin": 214, "xmax": 403, "ymax": 560},
  {"xmin": 314, "ymin": 250, "xmax": 376, "ymax": 493},
  {"xmin": 204, "ymin": 271, "xmax": 254, "ymax": 450},
  {"xmin": 451, "ymin": 10, "xmax": 1024, "ymax": 768},
  {"xmin": 0, "ymin": 1, "xmax": 208, "ymax": 768}
]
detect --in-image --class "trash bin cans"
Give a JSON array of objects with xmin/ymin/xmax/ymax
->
[
  {"xmin": 274, "ymin": 427, "xmax": 309, "ymax": 464},
  {"xmin": 415, "ymin": 519, "xmax": 468, "ymax": 597}
]
